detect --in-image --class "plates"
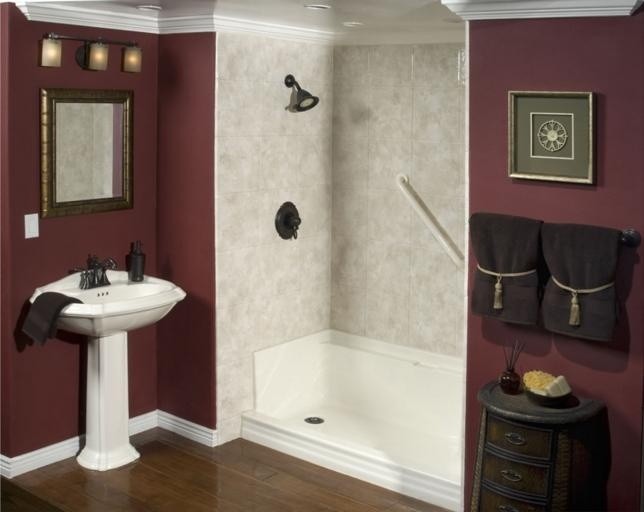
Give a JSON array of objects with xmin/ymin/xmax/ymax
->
[{"xmin": 522, "ymin": 386, "xmax": 573, "ymax": 407}]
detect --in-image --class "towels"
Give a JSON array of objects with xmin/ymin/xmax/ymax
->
[
  {"xmin": 19, "ymin": 291, "xmax": 82, "ymax": 345},
  {"xmin": 466, "ymin": 212, "xmax": 623, "ymax": 345}
]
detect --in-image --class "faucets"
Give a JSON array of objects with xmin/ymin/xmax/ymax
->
[{"xmin": 72, "ymin": 257, "xmax": 117, "ymax": 289}]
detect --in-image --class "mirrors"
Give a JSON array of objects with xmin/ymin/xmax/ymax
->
[{"xmin": 38, "ymin": 86, "xmax": 134, "ymax": 219}]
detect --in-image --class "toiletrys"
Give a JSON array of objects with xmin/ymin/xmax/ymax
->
[{"xmin": 129, "ymin": 240, "xmax": 146, "ymax": 281}]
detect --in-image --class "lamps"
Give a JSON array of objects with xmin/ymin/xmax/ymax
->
[{"xmin": 38, "ymin": 32, "xmax": 142, "ymax": 74}]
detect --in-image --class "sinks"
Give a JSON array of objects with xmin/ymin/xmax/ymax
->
[{"xmin": 29, "ymin": 269, "xmax": 187, "ymax": 336}]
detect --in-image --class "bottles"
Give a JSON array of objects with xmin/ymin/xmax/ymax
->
[{"xmin": 500, "ymin": 370, "xmax": 520, "ymax": 394}]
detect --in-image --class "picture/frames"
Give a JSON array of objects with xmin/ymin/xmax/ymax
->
[{"xmin": 507, "ymin": 89, "xmax": 595, "ymax": 186}]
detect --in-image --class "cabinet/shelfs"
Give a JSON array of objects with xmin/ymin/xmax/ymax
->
[{"xmin": 469, "ymin": 380, "xmax": 612, "ymax": 511}]
